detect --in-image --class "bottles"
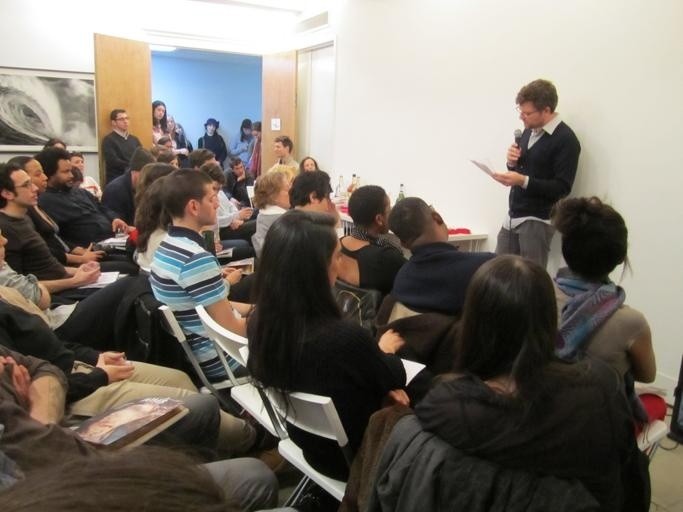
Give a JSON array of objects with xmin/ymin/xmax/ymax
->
[
  {"xmin": 334, "ymin": 175, "xmax": 344, "ymax": 197},
  {"xmin": 345, "ymin": 174, "xmax": 360, "ymax": 200},
  {"xmin": 395, "ymin": 184, "xmax": 405, "ymax": 203}
]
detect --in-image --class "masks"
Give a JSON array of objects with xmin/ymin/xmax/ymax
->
[{"xmin": 243, "ymin": 129, "xmax": 251, "ymax": 135}]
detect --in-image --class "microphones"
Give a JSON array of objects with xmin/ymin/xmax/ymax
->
[{"xmin": 514, "ymin": 129, "xmax": 521, "ymax": 166}]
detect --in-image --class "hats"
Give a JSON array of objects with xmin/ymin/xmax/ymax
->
[
  {"xmin": 129, "ymin": 146, "xmax": 157, "ymax": 171},
  {"xmin": 204, "ymin": 118, "xmax": 219, "ymax": 130},
  {"xmin": 242, "ymin": 119, "xmax": 252, "ymax": 128}
]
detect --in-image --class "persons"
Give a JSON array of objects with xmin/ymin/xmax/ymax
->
[
  {"xmin": 0, "ymin": 135, "xmax": 660, "ymax": 512},
  {"xmin": 164, "ymin": 114, "xmax": 188, "ymax": 169},
  {"xmin": 173, "ymin": 122, "xmax": 194, "ymax": 161},
  {"xmin": 152, "ymin": 100, "xmax": 168, "ymax": 145},
  {"xmin": 196, "ymin": 117, "xmax": 227, "ymax": 172},
  {"xmin": 492, "ymin": 78, "xmax": 581, "ymax": 272},
  {"xmin": 244, "ymin": 121, "xmax": 261, "ymax": 177},
  {"xmin": 100, "ymin": 108, "xmax": 143, "ymax": 186},
  {"xmin": 224, "ymin": 118, "xmax": 254, "ymax": 166}
]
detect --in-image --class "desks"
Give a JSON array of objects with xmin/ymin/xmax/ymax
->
[{"xmin": 333, "ymin": 202, "xmax": 489, "ymax": 254}]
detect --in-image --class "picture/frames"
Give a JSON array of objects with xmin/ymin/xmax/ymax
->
[{"xmin": 0, "ymin": 67, "xmax": 95, "ymax": 155}]
